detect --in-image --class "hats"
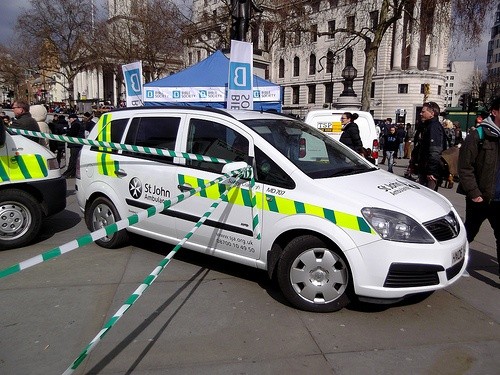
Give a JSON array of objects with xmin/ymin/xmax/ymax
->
[
  {"xmin": 84, "ymin": 112, "xmax": 91, "ymax": 117},
  {"xmin": 68, "ymin": 114, "xmax": 78, "ymax": 119}
]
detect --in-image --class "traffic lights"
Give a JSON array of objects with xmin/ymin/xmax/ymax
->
[
  {"xmin": 6, "ymin": 90, "xmax": 15, "ymax": 98},
  {"xmin": 460, "ymin": 92, "xmax": 484, "ymax": 112}
]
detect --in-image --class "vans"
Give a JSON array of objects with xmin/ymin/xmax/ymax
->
[{"xmin": 298, "ymin": 110, "xmax": 382, "ymax": 170}]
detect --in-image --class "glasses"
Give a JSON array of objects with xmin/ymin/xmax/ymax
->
[
  {"xmin": 428, "ymin": 102, "xmax": 433, "ymax": 109},
  {"xmin": 341, "ymin": 118, "xmax": 346, "ymax": 119},
  {"xmin": 12, "ymin": 107, "xmax": 22, "ymax": 109}
]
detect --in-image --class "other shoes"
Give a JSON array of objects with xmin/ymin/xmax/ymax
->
[{"xmin": 461, "ymin": 269, "xmax": 470, "ymax": 277}]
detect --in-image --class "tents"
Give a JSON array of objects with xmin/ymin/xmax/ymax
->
[{"xmin": 143, "ymin": 50, "xmax": 282, "ymax": 113}]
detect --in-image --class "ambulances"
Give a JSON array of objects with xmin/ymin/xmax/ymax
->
[
  {"xmin": 75, "ymin": 109, "xmax": 471, "ymax": 312},
  {"xmin": 0, "ymin": 116, "xmax": 67, "ymax": 250}
]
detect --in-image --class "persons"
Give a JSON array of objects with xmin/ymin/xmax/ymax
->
[
  {"xmin": 408, "ymin": 102, "xmax": 446, "ymax": 191},
  {"xmin": 380, "ymin": 108, "xmax": 490, "ymax": 173},
  {"xmin": 9, "ymin": 100, "xmax": 40, "ymax": 144},
  {"xmin": 0, "ymin": 109, "xmax": 98, "ymax": 179},
  {"xmin": 339, "ymin": 112, "xmax": 362, "ymax": 155},
  {"xmin": 456, "ymin": 98, "xmax": 500, "ymax": 278}
]
